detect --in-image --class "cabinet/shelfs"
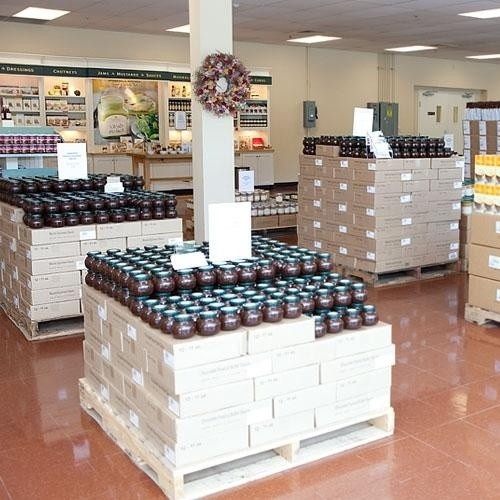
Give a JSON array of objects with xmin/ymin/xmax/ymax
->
[
  {"xmin": 134, "ymin": 155, "xmax": 192, "ymax": 194},
  {"xmin": 162, "ymin": 83, "xmax": 270, "ymax": 148},
  {"xmin": 42, "ymin": 77, "xmax": 88, "ymax": 128},
  {"xmin": 0, "ymin": 75, "xmax": 41, "ymax": 127},
  {"xmin": 0, "ymin": 133, "xmax": 87, "ymax": 168},
  {"xmin": 93, "ymin": 154, "xmax": 133, "ymax": 173},
  {"xmin": 242, "ymin": 152, "xmax": 274, "ymax": 186}
]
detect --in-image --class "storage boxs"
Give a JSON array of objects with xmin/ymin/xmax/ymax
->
[
  {"xmin": 470, "ymin": 211, "xmax": 500, "ymax": 249},
  {"xmin": 468, "ymin": 245, "xmax": 499, "ymax": 282},
  {"xmin": 467, "ymin": 274, "xmax": 500, "ymax": 315}
]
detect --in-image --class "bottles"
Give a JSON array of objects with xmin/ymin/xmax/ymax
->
[
  {"xmin": 118, "ymin": 266, "xmax": 137, "ymax": 288},
  {"xmin": 270, "ymin": 292, "xmax": 287, "ymax": 307},
  {"xmin": 176, "ymin": 289, "xmax": 192, "ymax": 302},
  {"xmin": 257, "ymin": 259, "xmax": 277, "ymax": 280},
  {"xmin": 333, "ymin": 305, "xmax": 347, "ymax": 319},
  {"xmin": 319, "ymin": 282, "xmax": 335, "ymax": 289},
  {"xmin": 108, "ymin": 282, "xmax": 116, "ymax": 297},
  {"xmin": 97, "ymin": 95, "xmax": 130, "ymax": 139},
  {"xmin": 275, "ymin": 280, "xmax": 288, "ymax": 293},
  {"xmin": 238, "ymin": 262, "xmax": 257, "ymax": 283},
  {"xmin": 319, "ymin": 271, "xmax": 331, "ymax": 282},
  {"xmin": 333, "ymin": 286, "xmax": 352, "ymax": 306},
  {"xmin": 1, "ymin": 105, "xmax": 5, "ymax": 120},
  {"xmin": 120, "ymin": 287, "xmax": 129, "ymax": 305},
  {"xmin": 102, "ymin": 280, "xmax": 110, "ymax": 293},
  {"xmin": 84, "ymin": 251, "xmax": 102, "ymax": 270},
  {"xmin": 284, "ymin": 287, "xmax": 298, "ymax": 295},
  {"xmin": 336, "ymin": 279, "xmax": 352, "ymax": 286},
  {"xmin": 325, "ymin": 311, "xmax": 344, "ymax": 333},
  {"xmin": 239, "ymin": 282, "xmax": 257, "ymax": 290},
  {"xmin": 169, "ymin": 101, "xmax": 192, "ymax": 128},
  {"xmin": 140, "ymin": 299, "xmax": 161, "ymax": 323},
  {"xmin": 91, "ymin": 254, "xmax": 107, "ymax": 273},
  {"xmin": 461, "ymin": 178, "xmax": 475, "ymax": 215},
  {"xmin": 137, "ymin": 240, "xmax": 209, "ymax": 273},
  {"xmin": 301, "ymin": 274, "xmax": 315, "ymax": 285},
  {"xmin": 159, "ymin": 309, "xmax": 180, "ymax": 334},
  {"xmin": 282, "ymin": 258, "xmax": 301, "ymax": 277},
  {"xmin": 0, "ymin": 172, "xmax": 178, "ymax": 228},
  {"xmin": 171, "ymin": 86, "xmax": 175, "ymax": 97},
  {"xmin": 5, "ymin": 104, "xmax": 12, "ymax": 120},
  {"xmin": 263, "ymin": 287, "xmax": 278, "ymax": 299},
  {"xmin": 272, "ymin": 254, "xmax": 288, "ymax": 274},
  {"xmin": 220, "ymin": 293, "xmax": 238, "ymax": 307},
  {"xmin": 184, "ymin": 305, "xmax": 204, "ymax": 323},
  {"xmin": 186, "ymin": 198, "xmax": 193, "ymax": 210},
  {"xmin": 148, "ymin": 304, "xmax": 169, "ymax": 329},
  {"xmin": 262, "ymin": 299, "xmax": 284, "ymax": 323},
  {"xmin": 99, "ymin": 257, "xmax": 115, "ymax": 277},
  {"xmin": 235, "ymin": 187, "xmax": 299, "ymax": 217},
  {"xmin": 164, "ymin": 295, "xmax": 182, "ymax": 310},
  {"xmin": 199, "ymin": 297, "xmax": 217, "ymax": 311},
  {"xmin": 208, "ymin": 302, "xmax": 225, "ymax": 318},
  {"xmin": 85, "ymin": 270, "xmax": 93, "ymax": 285},
  {"xmin": 315, "ymin": 288, "xmax": 335, "ymax": 309},
  {"xmin": 272, "ymin": 274, "xmax": 282, "ymax": 287},
  {"xmin": 220, "ymin": 285, "xmax": 235, "ymax": 293},
  {"xmin": 233, "ymin": 286, "xmax": 246, "ymax": 298},
  {"xmin": 93, "ymin": 274, "xmax": 103, "ymax": 290},
  {"xmin": 173, "ymin": 268, "xmax": 197, "ymax": 289},
  {"xmin": 130, "ymin": 296, "xmax": 150, "ymax": 316},
  {"xmin": 360, "ymin": 304, "xmax": 379, "ymax": 325},
  {"xmin": 350, "ymin": 302, "xmax": 364, "ymax": 313},
  {"xmin": 302, "ymin": 285, "xmax": 317, "ymax": 293},
  {"xmin": 316, "ymin": 252, "xmax": 334, "ymax": 272},
  {"xmin": 240, "ymin": 102, "xmax": 267, "ymax": 127},
  {"xmin": 229, "ymin": 298, "xmax": 247, "ymax": 316},
  {"xmin": 133, "ymin": 273, "xmax": 154, "ymax": 296},
  {"xmin": 175, "ymin": 300, "xmax": 194, "ymax": 314},
  {"xmin": 219, "ymin": 306, "xmax": 242, "ymax": 331},
  {"xmin": 283, "ymin": 276, "xmax": 297, "ymax": 288},
  {"xmin": 127, "ymin": 295, "xmax": 135, "ymax": 309},
  {"xmin": 256, "ymin": 283, "xmax": 270, "ymax": 295},
  {"xmin": 252, "ymin": 233, "xmax": 318, "ymax": 259},
  {"xmin": 170, "ymin": 313, "xmax": 196, "ymax": 339},
  {"xmin": 311, "ymin": 275, "xmax": 324, "ymax": 289},
  {"xmin": 126, "ymin": 269, "xmax": 145, "ymax": 294},
  {"xmin": 302, "ymin": 135, "xmax": 452, "ymax": 159},
  {"xmin": 298, "ymin": 255, "xmax": 318, "ymax": 275},
  {"xmin": 106, "ymin": 247, "xmax": 137, "ymax": 265},
  {"xmin": 315, "ymin": 309, "xmax": 330, "ymax": 320},
  {"xmin": 99, "ymin": 277, "xmax": 109, "ymax": 291},
  {"xmin": 113, "ymin": 284, "xmax": 122, "ymax": 301},
  {"xmin": 328, "ymin": 273, "xmax": 340, "ymax": 286},
  {"xmin": 152, "ymin": 270, "xmax": 175, "ymax": 293},
  {"xmin": 292, "ymin": 278, "xmax": 308, "ymax": 292},
  {"xmin": 195, "ymin": 311, "xmax": 221, "ymax": 336},
  {"xmin": 242, "ymin": 290, "xmax": 257, "ymax": 302},
  {"xmin": 199, "ymin": 286, "xmax": 214, "ymax": 297},
  {"xmin": 105, "ymin": 259, "xmax": 120, "ymax": 278},
  {"xmin": 312, "ymin": 315, "xmax": 327, "ymax": 338},
  {"xmin": 182, "ymin": 86, "xmax": 186, "ymax": 97},
  {"xmin": 155, "ymin": 292, "xmax": 171, "ymax": 305},
  {"xmin": 110, "ymin": 262, "xmax": 129, "ymax": 284},
  {"xmin": 212, "ymin": 289, "xmax": 226, "ymax": 303},
  {"xmin": 281, "ymin": 294, "xmax": 302, "ymax": 319},
  {"xmin": 350, "ymin": 283, "xmax": 368, "ymax": 303},
  {"xmin": 342, "ymin": 308, "xmax": 362, "ymax": 330},
  {"xmin": 249, "ymin": 294, "xmax": 267, "ymax": 311},
  {"xmin": 195, "ymin": 265, "xmax": 217, "ymax": 286},
  {"xmin": 189, "ymin": 292, "xmax": 205, "ymax": 306},
  {"xmin": 217, "ymin": 264, "xmax": 239, "ymax": 285},
  {"xmin": 88, "ymin": 273, "xmax": 97, "ymax": 286},
  {"xmin": 240, "ymin": 302, "xmax": 264, "ymax": 326},
  {"xmin": 297, "ymin": 291, "xmax": 316, "ymax": 312}
]
